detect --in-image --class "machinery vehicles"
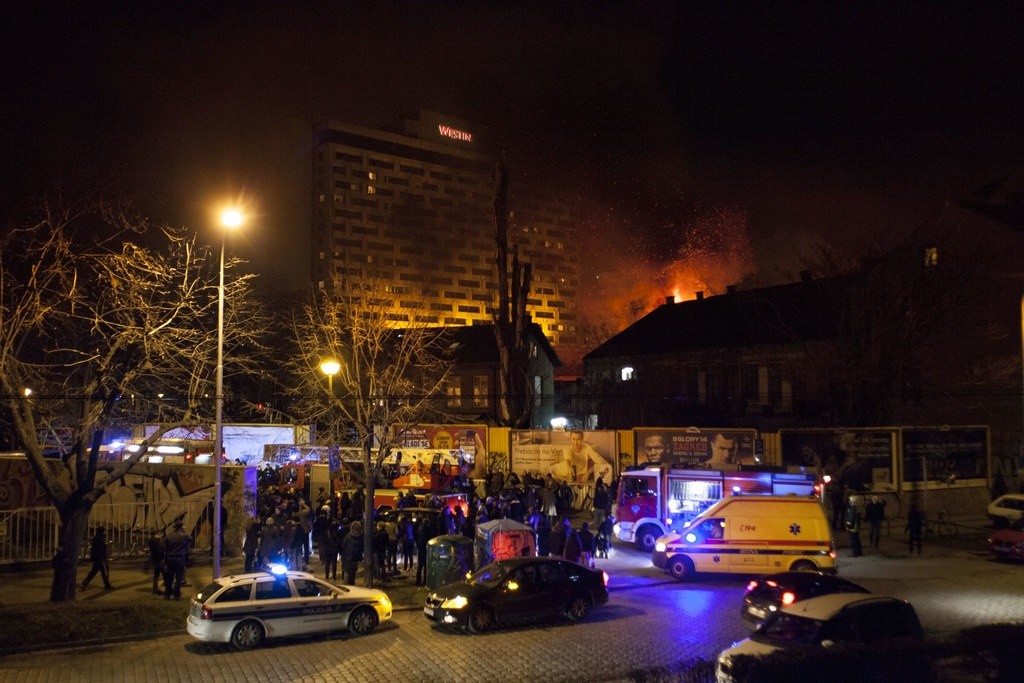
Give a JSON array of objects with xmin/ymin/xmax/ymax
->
[{"xmin": 263, "ymin": 442, "xmax": 474, "ymax": 524}]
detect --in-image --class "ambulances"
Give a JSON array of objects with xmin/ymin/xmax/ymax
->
[{"xmin": 653, "ymin": 493, "xmax": 836, "ymax": 581}]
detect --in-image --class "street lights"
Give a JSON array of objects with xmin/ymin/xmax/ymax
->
[
  {"xmin": 320, "ymin": 357, "xmax": 341, "ymax": 502},
  {"xmin": 212, "ymin": 194, "xmax": 253, "ymax": 578}
]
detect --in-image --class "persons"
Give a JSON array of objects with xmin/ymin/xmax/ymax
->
[
  {"xmin": 864, "ymin": 496, "xmax": 885, "ymax": 549},
  {"xmin": 643, "ymin": 431, "xmax": 670, "ymax": 462},
  {"xmin": 235, "ymin": 458, "xmax": 246, "ymax": 465},
  {"xmin": 594, "ymin": 473, "xmax": 619, "ymax": 530},
  {"xmin": 904, "ymin": 504, "xmax": 922, "ymax": 554},
  {"xmin": 831, "ymin": 480, "xmax": 847, "ymax": 531},
  {"xmin": 598, "ymin": 515, "xmax": 615, "ymax": 559},
  {"xmin": 257, "ymin": 464, "xmax": 274, "ymax": 481},
  {"xmin": 148, "ymin": 530, "xmax": 175, "ymax": 594},
  {"xmin": 578, "ymin": 522, "xmax": 595, "ymax": 568},
  {"xmin": 80, "ymin": 527, "xmax": 115, "ymax": 591},
  {"xmin": 242, "ymin": 473, "xmax": 582, "ymax": 585},
  {"xmin": 165, "ymin": 520, "xmax": 193, "ymax": 601},
  {"xmin": 208, "ymin": 507, "xmax": 228, "ymax": 549},
  {"xmin": 845, "ymin": 496, "xmax": 862, "ymax": 557},
  {"xmin": 704, "ymin": 433, "xmax": 739, "ymax": 464},
  {"xmin": 564, "ymin": 431, "xmax": 606, "ymax": 482}
]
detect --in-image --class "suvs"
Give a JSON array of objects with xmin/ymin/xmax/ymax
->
[{"xmin": 715, "ymin": 592, "xmax": 923, "ymax": 683}]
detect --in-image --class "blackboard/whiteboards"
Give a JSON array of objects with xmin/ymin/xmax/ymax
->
[
  {"xmin": 900, "ymin": 425, "xmax": 991, "ymax": 491},
  {"xmin": 778, "ymin": 428, "xmax": 899, "ymax": 492}
]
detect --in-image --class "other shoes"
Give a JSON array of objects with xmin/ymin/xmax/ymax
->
[
  {"xmin": 858, "ymin": 550, "xmax": 861, "ymax": 555},
  {"xmin": 180, "ymin": 580, "xmax": 192, "ymax": 587},
  {"xmin": 80, "ymin": 584, "xmax": 88, "ymax": 592},
  {"xmin": 165, "ymin": 592, "xmax": 170, "ymax": 599},
  {"xmin": 152, "ymin": 589, "xmax": 164, "ymax": 596},
  {"xmin": 173, "ymin": 596, "xmax": 179, "ymax": 600},
  {"xmin": 847, "ymin": 553, "xmax": 858, "ymax": 557},
  {"xmin": 105, "ymin": 587, "xmax": 115, "ymax": 590}
]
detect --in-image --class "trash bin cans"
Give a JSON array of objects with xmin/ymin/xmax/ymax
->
[{"xmin": 426, "ymin": 535, "xmax": 474, "ymax": 590}]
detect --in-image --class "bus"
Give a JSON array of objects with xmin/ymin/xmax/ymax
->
[{"xmin": 102, "ymin": 434, "xmax": 226, "ymax": 466}]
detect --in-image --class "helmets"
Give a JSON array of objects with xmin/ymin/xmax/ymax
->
[{"xmin": 849, "ymin": 496, "xmax": 858, "ymax": 502}]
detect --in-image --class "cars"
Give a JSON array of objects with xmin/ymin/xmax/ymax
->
[
  {"xmin": 188, "ymin": 562, "xmax": 393, "ymax": 651},
  {"xmin": 739, "ymin": 569, "xmax": 871, "ymax": 634},
  {"xmin": 985, "ymin": 494, "xmax": 1024, "ymax": 529},
  {"xmin": 424, "ymin": 555, "xmax": 610, "ymax": 634},
  {"xmin": 987, "ymin": 515, "xmax": 1024, "ymax": 564}
]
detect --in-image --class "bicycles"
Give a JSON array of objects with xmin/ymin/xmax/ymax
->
[{"xmin": 903, "ymin": 509, "xmax": 960, "ymax": 542}]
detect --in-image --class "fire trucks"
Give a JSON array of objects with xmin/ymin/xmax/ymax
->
[{"xmin": 612, "ymin": 463, "xmax": 830, "ymax": 558}]
